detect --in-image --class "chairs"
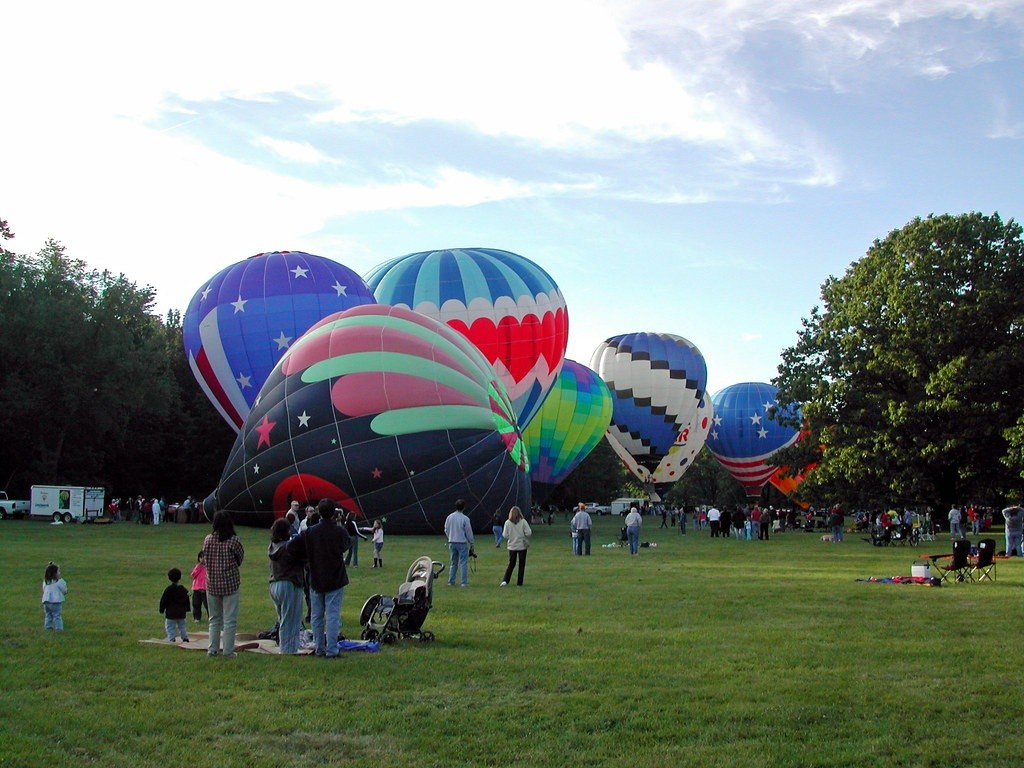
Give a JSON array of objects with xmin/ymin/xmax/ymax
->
[{"xmin": 933, "ymin": 538, "xmax": 996, "ymax": 585}]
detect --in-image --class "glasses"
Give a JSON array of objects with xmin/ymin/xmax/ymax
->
[
  {"xmin": 307, "ymin": 510, "xmax": 316, "ymax": 513},
  {"xmin": 292, "ymin": 504, "xmax": 299, "ymax": 507}
]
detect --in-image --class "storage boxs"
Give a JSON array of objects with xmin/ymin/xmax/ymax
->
[{"xmin": 911, "ymin": 564, "xmax": 930, "ymax": 578}]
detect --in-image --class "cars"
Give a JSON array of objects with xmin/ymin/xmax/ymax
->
[{"xmin": 573, "ymin": 502, "xmax": 611, "ymax": 516}]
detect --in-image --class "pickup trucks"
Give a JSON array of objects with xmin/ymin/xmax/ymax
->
[{"xmin": 0, "ymin": 491, "xmax": 33, "ymax": 521}]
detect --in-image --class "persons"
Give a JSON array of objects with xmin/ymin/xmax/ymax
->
[
  {"xmin": 286, "ymin": 498, "xmax": 353, "ymax": 658},
  {"xmin": 444, "ymin": 500, "xmax": 476, "ymax": 586},
  {"xmin": 948, "ymin": 504, "xmax": 970, "ymax": 540},
  {"xmin": 968, "ymin": 504, "xmax": 995, "ymax": 536},
  {"xmin": 286, "ymin": 500, "xmax": 300, "ymax": 537},
  {"xmin": 183, "ymin": 496, "xmax": 204, "ymax": 524},
  {"xmin": 500, "ymin": 506, "xmax": 532, "ymax": 587},
  {"xmin": 268, "ymin": 518, "xmax": 304, "ymax": 654},
  {"xmin": 202, "ymin": 509, "xmax": 244, "ymax": 657},
  {"xmin": 42, "ymin": 560, "xmax": 68, "ymax": 631},
  {"xmin": 570, "ymin": 502, "xmax": 592, "ymax": 556},
  {"xmin": 305, "ymin": 513, "xmax": 321, "ymax": 623},
  {"xmin": 300, "ymin": 505, "xmax": 315, "ymax": 531},
  {"xmin": 492, "ymin": 509, "xmax": 503, "ymax": 548},
  {"xmin": 126, "ymin": 494, "xmax": 169, "ymax": 526},
  {"xmin": 335, "ymin": 506, "xmax": 368, "ymax": 566},
  {"xmin": 625, "ymin": 507, "xmax": 642, "ymax": 553},
  {"xmin": 660, "ymin": 505, "xmax": 706, "ymax": 535},
  {"xmin": 1002, "ymin": 504, "xmax": 1024, "ymax": 557},
  {"xmin": 358, "ymin": 520, "xmax": 383, "ymax": 568},
  {"xmin": 107, "ymin": 497, "xmax": 123, "ymax": 520},
  {"xmin": 160, "ymin": 568, "xmax": 191, "ymax": 642},
  {"xmin": 192, "ymin": 549, "xmax": 209, "ymax": 623},
  {"xmin": 708, "ymin": 504, "xmax": 938, "ymax": 546}
]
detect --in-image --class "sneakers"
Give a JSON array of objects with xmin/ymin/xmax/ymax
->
[
  {"xmin": 500, "ymin": 582, "xmax": 507, "ymax": 587},
  {"xmin": 448, "ymin": 583, "xmax": 455, "ymax": 586},
  {"xmin": 461, "ymin": 584, "xmax": 467, "ymax": 587}
]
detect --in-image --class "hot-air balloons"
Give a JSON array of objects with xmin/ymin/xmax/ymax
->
[
  {"xmin": 768, "ymin": 419, "xmax": 838, "ymax": 513},
  {"xmin": 182, "ymin": 251, "xmax": 378, "ymax": 436},
  {"xmin": 646, "ymin": 391, "xmax": 713, "ymax": 504},
  {"xmin": 174, "ymin": 303, "xmax": 554, "ymax": 542},
  {"xmin": 588, "ymin": 332, "xmax": 709, "ymax": 498},
  {"xmin": 519, "ymin": 358, "xmax": 615, "ymax": 523},
  {"xmin": 359, "ymin": 245, "xmax": 569, "ymax": 435},
  {"xmin": 706, "ymin": 382, "xmax": 805, "ymax": 511}
]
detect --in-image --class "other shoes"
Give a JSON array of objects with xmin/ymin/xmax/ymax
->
[
  {"xmin": 183, "ymin": 638, "xmax": 189, "ymax": 643},
  {"xmin": 170, "ymin": 638, "xmax": 176, "ymax": 643},
  {"xmin": 207, "ymin": 652, "xmax": 219, "ymax": 656},
  {"xmin": 224, "ymin": 652, "xmax": 237, "ymax": 659}
]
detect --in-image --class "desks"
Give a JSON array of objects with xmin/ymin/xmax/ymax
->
[{"xmin": 919, "ymin": 553, "xmax": 1009, "ymax": 585}]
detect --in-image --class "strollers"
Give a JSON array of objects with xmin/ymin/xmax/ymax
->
[{"xmin": 360, "ymin": 556, "xmax": 446, "ymax": 646}]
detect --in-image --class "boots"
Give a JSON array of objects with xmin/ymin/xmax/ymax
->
[{"xmin": 371, "ymin": 558, "xmax": 382, "ymax": 568}]
